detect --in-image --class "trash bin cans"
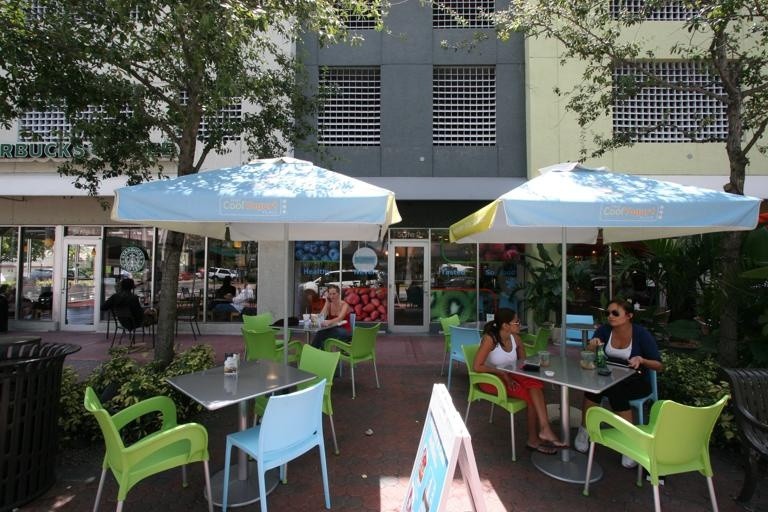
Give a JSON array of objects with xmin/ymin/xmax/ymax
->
[{"xmin": 0, "ymin": 336, "xmax": 81, "ymax": 511}]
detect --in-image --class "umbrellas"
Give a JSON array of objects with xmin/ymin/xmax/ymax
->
[
  {"xmin": 446, "ymin": 160, "xmax": 763, "ymax": 357},
  {"xmin": 111, "ymin": 155, "xmax": 404, "ymax": 365}
]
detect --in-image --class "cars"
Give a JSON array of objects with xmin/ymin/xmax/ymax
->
[
  {"xmin": 590, "ymin": 276, "xmax": 609, "ymax": 293},
  {"xmin": 178, "ymin": 267, "xmax": 240, "ymax": 283},
  {"xmin": 0, "ymin": 261, "xmax": 134, "ymax": 308},
  {"xmin": 438, "ymin": 263, "xmax": 497, "ymax": 291}
]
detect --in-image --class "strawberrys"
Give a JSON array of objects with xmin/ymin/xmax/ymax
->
[{"xmin": 344, "ymin": 288, "xmax": 388, "ymax": 322}]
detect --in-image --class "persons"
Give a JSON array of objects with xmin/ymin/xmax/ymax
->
[
  {"xmin": 209, "ymin": 274, "xmax": 237, "ymax": 309},
  {"xmin": 405, "ymin": 281, "xmax": 423, "ymax": 310},
  {"xmin": 573, "ymin": 297, "xmax": 666, "ymax": 469},
  {"xmin": 309, "ymin": 283, "xmax": 351, "ymax": 351},
  {"xmin": 472, "ymin": 305, "xmax": 567, "ymax": 454},
  {"xmin": 99, "ymin": 277, "xmax": 157, "ymax": 330},
  {"xmin": 213, "ymin": 278, "xmax": 256, "ymax": 314},
  {"xmin": 0, "ymin": 283, "xmax": 10, "ymax": 334}
]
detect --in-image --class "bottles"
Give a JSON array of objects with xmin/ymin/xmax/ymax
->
[
  {"xmin": 223, "ymin": 350, "xmax": 240, "ymax": 375},
  {"xmin": 596, "ymin": 342, "xmax": 608, "ymax": 369},
  {"xmin": 298, "ymin": 313, "xmax": 324, "ymax": 331}
]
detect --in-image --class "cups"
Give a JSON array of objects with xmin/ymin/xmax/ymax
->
[
  {"xmin": 579, "ymin": 350, "xmax": 595, "ymax": 370},
  {"xmin": 539, "ymin": 349, "xmax": 552, "ymax": 367}
]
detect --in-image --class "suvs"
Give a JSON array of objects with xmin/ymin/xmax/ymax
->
[{"xmin": 300, "ymin": 269, "xmax": 384, "ymax": 305}]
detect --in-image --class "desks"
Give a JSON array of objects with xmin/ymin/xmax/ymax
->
[{"xmin": 165, "ymin": 359, "xmax": 318, "ymax": 509}]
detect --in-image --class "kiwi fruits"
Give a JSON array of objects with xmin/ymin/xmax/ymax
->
[{"xmin": 430, "ymin": 289, "xmax": 494, "ymax": 324}]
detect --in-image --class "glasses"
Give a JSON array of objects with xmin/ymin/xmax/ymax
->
[
  {"xmin": 508, "ymin": 319, "xmax": 520, "ymax": 326},
  {"xmin": 603, "ymin": 310, "xmax": 619, "ymax": 317}
]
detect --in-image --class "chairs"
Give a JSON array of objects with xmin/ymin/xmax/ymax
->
[
  {"xmin": 80, "ymin": 386, "xmax": 218, "ymax": 512},
  {"xmin": 220, "ymin": 311, "xmax": 380, "ymax": 512},
  {"xmin": 104, "ymin": 288, "xmax": 205, "ymax": 351},
  {"xmin": 197, "ymin": 276, "xmax": 258, "ymax": 321}
]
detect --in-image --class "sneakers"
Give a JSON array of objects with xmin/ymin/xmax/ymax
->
[
  {"xmin": 621, "ymin": 454, "xmax": 637, "ymax": 469},
  {"xmin": 574, "ymin": 425, "xmax": 588, "ymax": 453}
]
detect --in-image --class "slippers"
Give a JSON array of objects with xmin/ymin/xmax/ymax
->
[
  {"xmin": 538, "ymin": 439, "xmax": 570, "ymax": 449},
  {"xmin": 526, "ymin": 443, "xmax": 558, "ymax": 455}
]
